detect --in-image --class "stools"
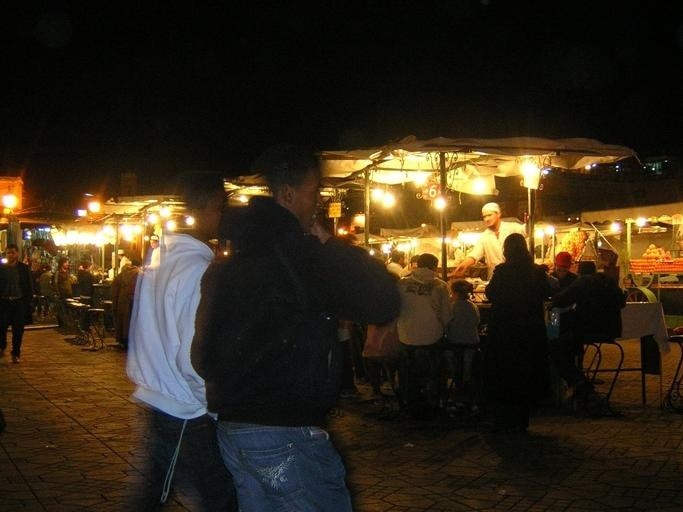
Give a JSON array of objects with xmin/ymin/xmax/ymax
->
[
  {"xmin": 369, "ymin": 334, "xmax": 624, "ymax": 423},
  {"xmin": 33, "ymin": 293, "xmax": 113, "ymax": 353},
  {"xmin": 667, "ymin": 334, "xmax": 683, "ymax": 415}
]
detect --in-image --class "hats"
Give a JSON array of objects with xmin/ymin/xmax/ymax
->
[
  {"xmin": 555, "ymin": 251, "xmax": 572, "ymax": 268},
  {"xmin": 481, "ymin": 202, "xmax": 501, "ymax": 214}
]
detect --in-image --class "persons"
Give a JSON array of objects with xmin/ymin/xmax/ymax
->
[
  {"xmin": 0, "ymin": 243, "xmax": 36, "ymax": 364},
  {"xmin": 188, "ymin": 146, "xmax": 404, "ymax": 511},
  {"xmin": 122, "ymin": 173, "xmax": 241, "ymax": 511},
  {"xmin": 336, "ymin": 234, "xmax": 626, "ymax": 435},
  {"xmin": 450, "ymin": 201, "xmax": 530, "ymax": 280},
  {"xmin": 25, "ymin": 256, "xmax": 142, "ymax": 352}
]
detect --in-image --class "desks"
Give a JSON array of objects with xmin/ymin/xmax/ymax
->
[{"xmin": 474, "ymin": 301, "xmax": 666, "ymax": 409}]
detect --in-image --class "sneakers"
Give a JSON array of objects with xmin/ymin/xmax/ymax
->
[{"xmin": 11, "ymin": 356, "xmax": 20, "ymax": 364}]
